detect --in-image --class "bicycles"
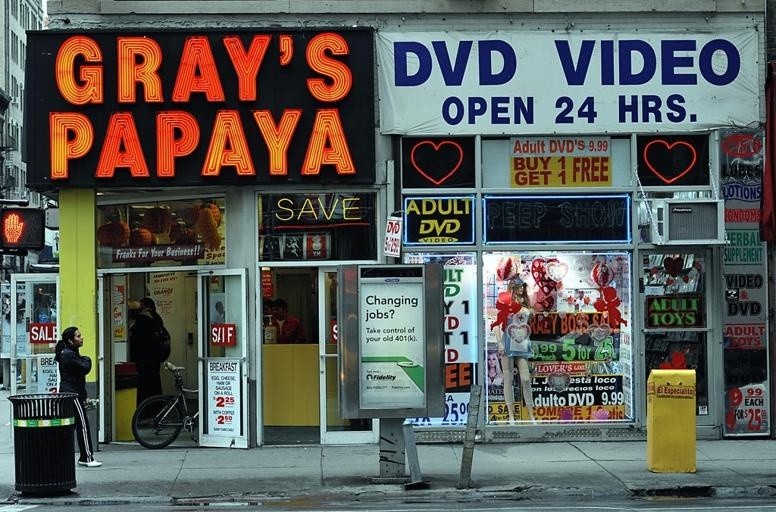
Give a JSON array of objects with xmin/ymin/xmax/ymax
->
[{"xmin": 131, "ymin": 361, "xmax": 198, "ymax": 449}]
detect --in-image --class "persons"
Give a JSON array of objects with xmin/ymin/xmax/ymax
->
[
  {"xmin": 210, "ymin": 301, "xmax": 226, "ymax": 323},
  {"xmin": 16, "ymin": 292, "xmax": 25, "ymax": 309},
  {"xmin": 127, "ymin": 295, "xmax": 169, "ymax": 429},
  {"xmin": 269, "ymin": 299, "xmax": 303, "ymax": 343},
  {"xmin": 53, "ymin": 326, "xmax": 102, "ymax": 467},
  {"xmin": 263, "ymin": 298, "xmax": 277, "ymax": 327},
  {"xmin": 1, "ymin": 293, "xmax": 10, "ymax": 316},
  {"xmin": 491, "ymin": 276, "xmax": 535, "ymax": 422}
]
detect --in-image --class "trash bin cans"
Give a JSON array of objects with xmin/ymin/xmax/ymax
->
[
  {"xmin": 8, "ymin": 393, "xmax": 79, "ymax": 498},
  {"xmin": 116, "ymin": 374, "xmax": 142, "ymax": 442},
  {"xmin": 645, "ymin": 368, "xmax": 698, "ymax": 472}
]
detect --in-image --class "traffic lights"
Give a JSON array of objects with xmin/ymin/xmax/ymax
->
[{"xmin": 0, "ymin": 207, "xmax": 45, "ymax": 250}]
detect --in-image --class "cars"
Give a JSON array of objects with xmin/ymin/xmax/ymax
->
[{"xmin": 522, "ymin": 327, "xmax": 621, "ymax": 362}]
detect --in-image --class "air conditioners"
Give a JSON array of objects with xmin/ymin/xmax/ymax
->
[{"xmin": 648, "ymin": 198, "xmax": 727, "ymax": 247}]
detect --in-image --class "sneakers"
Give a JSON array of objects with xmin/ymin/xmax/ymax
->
[{"xmin": 79, "ymin": 459, "xmax": 102, "ymax": 467}]
